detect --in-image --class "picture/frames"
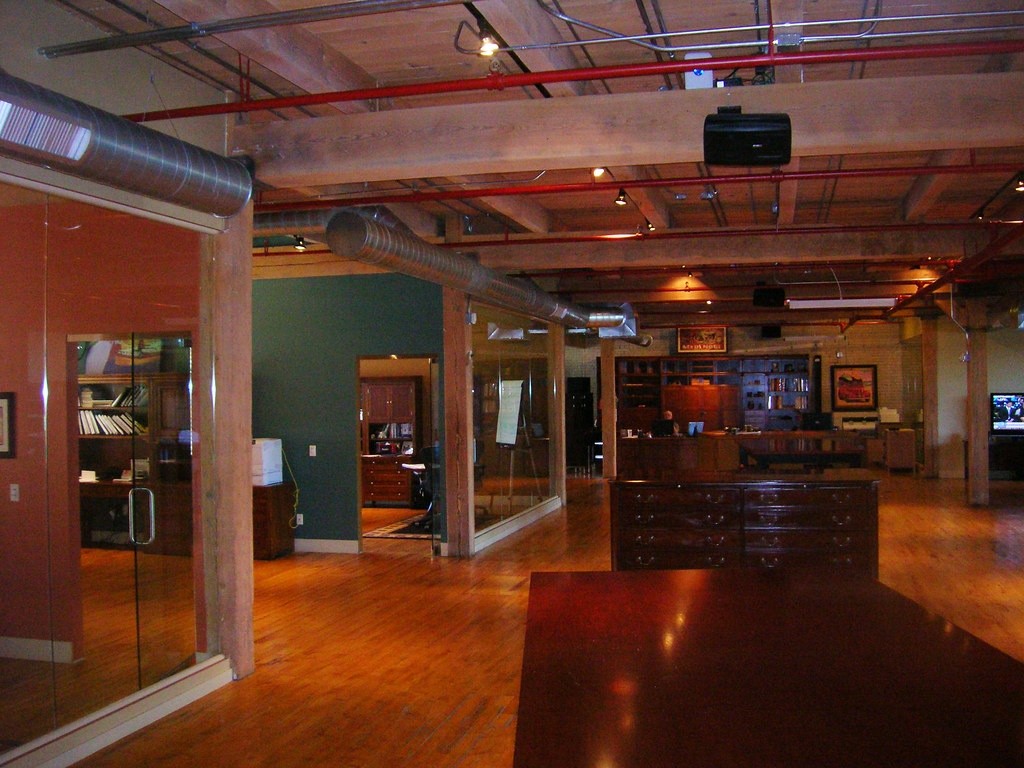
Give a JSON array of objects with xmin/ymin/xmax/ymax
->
[
  {"xmin": 0, "ymin": 392, "xmax": 18, "ymax": 459},
  {"xmin": 830, "ymin": 365, "xmax": 878, "ymax": 411},
  {"xmin": 676, "ymin": 326, "xmax": 727, "ymax": 354}
]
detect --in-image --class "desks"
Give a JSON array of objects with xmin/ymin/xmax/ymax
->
[
  {"xmin": 617, "ymin": 430, "xmax": 869, "ymax": 481},
  {"xmin": 962, "ymin": 435, "xmax": 1023, "ymax": 480},
  {"xmin": 879, "ymin": 427, "xmax": 918, "ymax": 476}
]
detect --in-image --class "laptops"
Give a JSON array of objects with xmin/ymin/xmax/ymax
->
[
  {"xmin": 688, "ymin": 421, "xmax": 705, "ymax": 437},
  {"xmin": 651, "ymin": 420, "xmax": 674, "ymax": 437}
]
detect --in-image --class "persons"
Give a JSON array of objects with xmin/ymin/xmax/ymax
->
[
  {"xmin": 999, "ymin": 401, "xmax": 1013, "ymax": 417},
  {"xmin": 663, "ymin": 411, "xmax": 679, "ymax": 432}
]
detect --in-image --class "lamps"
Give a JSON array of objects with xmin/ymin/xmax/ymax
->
[
  {"xmin": 751, "ymin": 287, "xmax": 787, "ymax": 306},
  {"xmin": 704, "ymin": 107, "xmax": 792, "ymax": 169},
  {"xmin": 687, "ymin": 272, "xmax": 692, "ymax": 278},
  {"xmin": 476, "ymin": 13, "xmax": 497, "ymax": 56},
  {"xmin": 644, "ymin": 219, "xmax": 655, "ymax": 233},
  {"xmin": 616, "ymin": 186, "xmax": 628, "ymax": 204},
  {"xmin": 292, "ymin": 237, "xmax": 307, "ymax": 253},
  {"xmin": 952, "ymin": 282, "xmax": 971, "ymax": 362},
  {"xmin": 635, "ymin": 224, "xmax": 644, "ymax": 236},
  {"xmin": 761, "ymin": 322, "xmax": 781, "ymax": 339},
  {"xmin": 787, "ymin": 297, "xmax": 898, "ymax": 309}
]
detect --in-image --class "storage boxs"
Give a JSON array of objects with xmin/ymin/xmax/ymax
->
[{"xmin": 253, "ymin": 437, "xmax": 283, "ymax": 486}]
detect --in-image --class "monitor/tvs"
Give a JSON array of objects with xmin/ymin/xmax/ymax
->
[
  {"xmin": 989, "ymin": 393, "xmax": 1024, "ymax": 436},
  {"xmin": 803, "ymin": 413, "xmax": 833, "ymax": 431}
]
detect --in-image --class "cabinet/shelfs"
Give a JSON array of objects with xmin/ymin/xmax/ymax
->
[
  {"xmin": 606, "ymin": 476, "xmax": 881, "ymax": 578},
  {"xmin": 596, "ymin": 357, "xmax": 743, "ymax": 434},
  {"xmin": 77, "ymin": 332, "xmax": 294, "ymax": 556},
  {"xmin": 362, "ymin": 377, "xmax": 426, "ymax": 508}
]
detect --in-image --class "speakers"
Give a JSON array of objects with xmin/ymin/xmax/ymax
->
[
  {"xmin": 753, "ymin": 287, "xmax": 785, "ymax": 308},
  {"xmin": 762, "ymin": 324, "xmax": 782, "ymax": 338},
  {"xmin": 703, "ymin": 112, "xmax": 792, "ymax": 167}
]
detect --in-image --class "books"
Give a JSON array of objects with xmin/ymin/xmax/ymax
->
[
  {"xmin": 768, "ymin": 378, "xmax": 809, "ymax": 409},
  {"xmin": 377, "ymin": 423, "xmax": 411, "ymax": 438},
  {"xmin": 78, "ymin": 384, "xmax": 149, "ymax": 435},
  {"xmin": 79, "ymin": 459, "xmax": 133, "ymax": 482}
]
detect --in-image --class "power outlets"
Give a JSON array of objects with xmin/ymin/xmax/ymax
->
[
  {"xmin": 296, "ymin": 513, "xmax": 304, "ymax": 526},
  {"xmin": 10, "ymin": 483, "xmax": 20, "ymax": 502}
]
